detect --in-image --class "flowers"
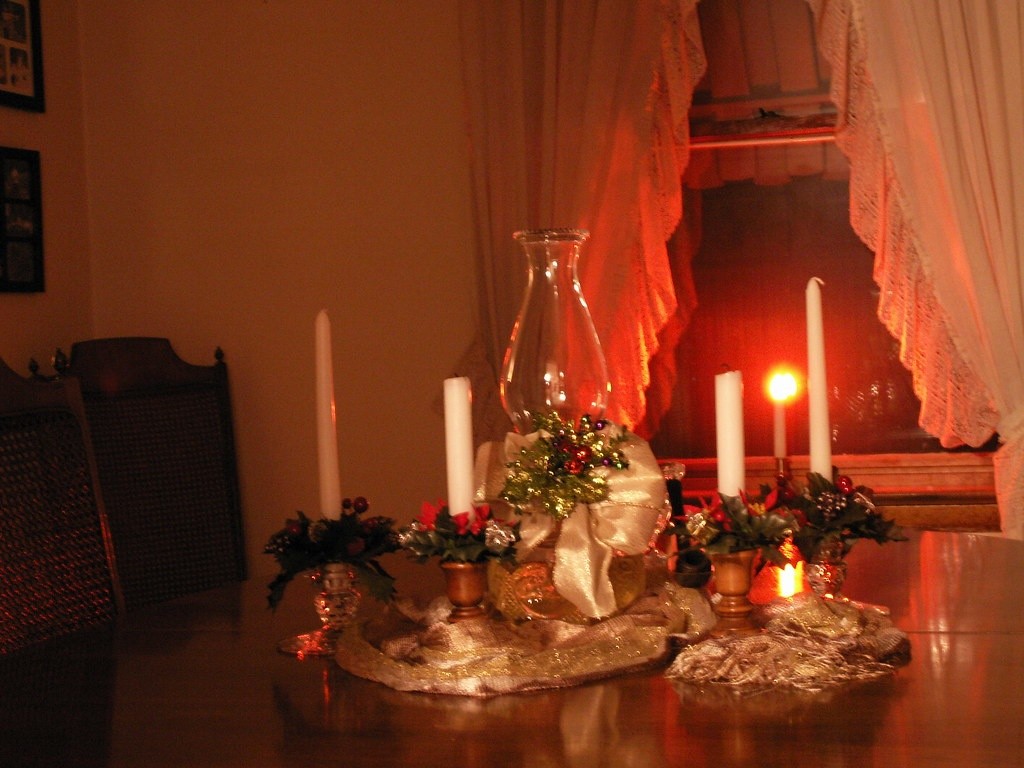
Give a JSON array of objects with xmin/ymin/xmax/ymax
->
[
  {"xmin": 402, "ymin": 501, "xmax": 517, "ymax": 564},
  {"xmin": 263, "ymin": 496, "xmax": 408, "ymax": 607},
  {"xmin": 672, "ymin": 469, "xmax": 900, "ymax": 582}
]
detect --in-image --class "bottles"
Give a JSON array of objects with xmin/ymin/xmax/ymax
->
[{"xmin": 497, "ymin": 228, "xmax": 608, "ymax": 436}]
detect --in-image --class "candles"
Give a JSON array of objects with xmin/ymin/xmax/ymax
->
[
  {"xmin": 443, "ymin": 373, "xmax": 474, "ymax": 523},
  {"xmin": 807, "ymin": 277, "xmax": 834, "ymax": 485},
  {"xmin": 316, "ymin": 308, "xmax": 342, "ymax": 519},
  {"xmin": 715, "ymin": 365, "xmax": 746, "ymax": 497},
  {"xmin": 774, "ymin": 403, "xmax": 786, "ymax": 462}
]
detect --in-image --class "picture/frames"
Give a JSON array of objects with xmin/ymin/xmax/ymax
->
[
  {"xmin": 0, "ymin": 0, "xmax": 45, "ymax": 113},
  {"xmin": 0, "ymin": 146, "xmax": 46, "ymax": 293}
]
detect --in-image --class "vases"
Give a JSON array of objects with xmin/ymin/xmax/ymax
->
[{"xmin": 500, "ymin": 229, "xmax": 648, "ymax": 625}]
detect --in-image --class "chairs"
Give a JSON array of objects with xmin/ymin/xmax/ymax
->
[
  {"xmin": 27, "ymin": 331, "xmax": 249, "ymax": 619},
  {"xmin": 0, "ymin": 348, "xmax": 124, "ymax": 661}
]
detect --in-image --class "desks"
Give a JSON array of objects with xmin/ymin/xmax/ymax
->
[{"xmin": 0, "ymin": 533, "xmax": 1024, "ymax": 768}]
